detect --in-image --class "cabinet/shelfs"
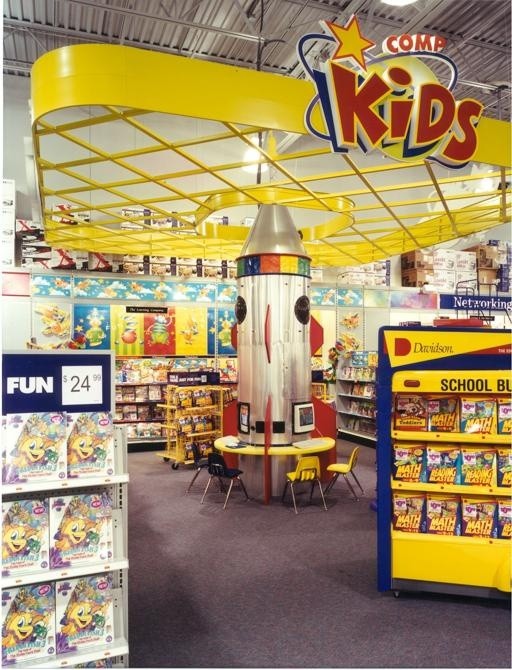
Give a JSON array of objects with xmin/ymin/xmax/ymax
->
[
  {"xmin": 3, "ymin": 350, "xmax": 223, "ymax": 668},
  {"xmin": 337, "ymin": 326, "xmax": 511, "ymax": 599}
]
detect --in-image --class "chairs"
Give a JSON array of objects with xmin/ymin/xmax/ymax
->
[
  {"xmin": 186, "ymin": 442, "xmax": 227, "ymax": 493},
  {"xmin": 321, "ymin": 446, "xmax": 365, "ymax": 501},
  {"xmin": 201, "ymin": 453, "xmax": 249, "ymax": 509},
  {"xmin": 281, "ymin": 457, "xmax": 328, "ymax": 514}
]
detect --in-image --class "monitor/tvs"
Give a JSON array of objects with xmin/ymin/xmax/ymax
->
[{"xmin": 236, "ymin": 402, "xmax": 251, "ymax": 434}]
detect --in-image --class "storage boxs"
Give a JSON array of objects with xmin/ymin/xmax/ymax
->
[{"xmin": 2, "ymin": 178, "xmax": 512, "ymax": 298}]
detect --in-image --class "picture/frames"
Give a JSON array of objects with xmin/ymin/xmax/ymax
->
[{"xmin": 238, "ymin": 402, "xmax": 250, "ymax": 435}]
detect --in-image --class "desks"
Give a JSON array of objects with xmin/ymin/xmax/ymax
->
[{"xmin": 213, "ymin": 436, "xmax": 336, "ymax": 459}]
